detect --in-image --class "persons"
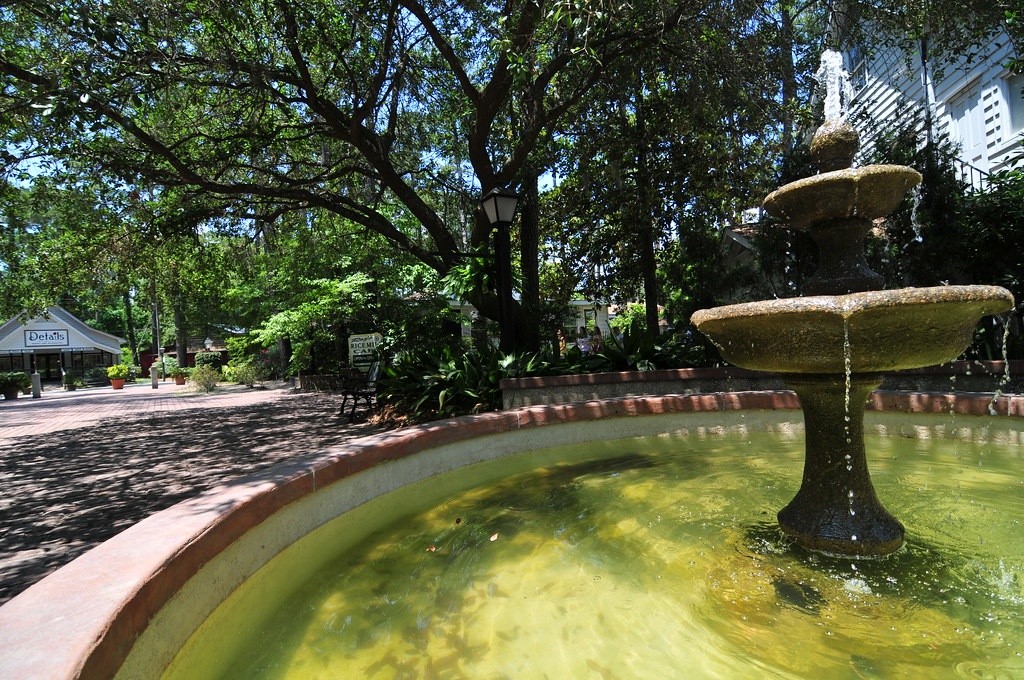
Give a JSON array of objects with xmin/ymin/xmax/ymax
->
[
  {"xmin": 557, "ymin": 330, "xmax": 567, "ymax": 354},
  {"xmin": 576, "ymin": 326, "xmax": 592, "ymax": 357},
  {"xmin": 592, "ymin": 326, "xmax": 602, "ymax": 351},
  {"xmin": 612, "ymin": 326, "xmax": 624, "ymax": 347}
]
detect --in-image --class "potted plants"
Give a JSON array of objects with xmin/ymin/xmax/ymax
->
[
  {"xmin": 170, "ymin": 365, "xmax": 189, "ymax": 385},
  {"xmin": 0, "ymin": 372, "xmax": 32, "ymax": 399},
  {"xmin": 64, "ymin": 368, "xmax": 83, "ymax": 391},
  {"xmin": 107, "ymin": 364, "xmax": 129, "ymax": 389}
]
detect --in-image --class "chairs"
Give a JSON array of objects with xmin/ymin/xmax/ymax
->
[{"xmin": 339, "ymin": 366, "xmax": 381, "ymax": 417}]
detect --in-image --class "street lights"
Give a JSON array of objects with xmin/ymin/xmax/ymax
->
[{"xmin": 481, "ymin": 180, "xmax": 521, "ymax": 357}]
